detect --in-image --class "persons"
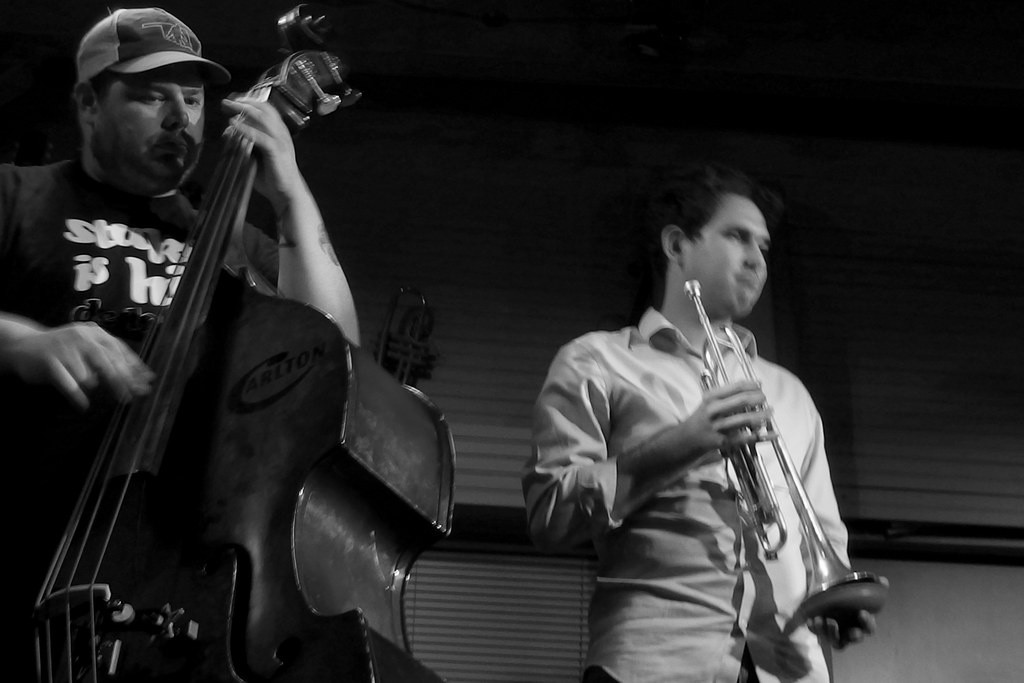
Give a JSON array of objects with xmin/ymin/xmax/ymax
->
[
  {"xmin": 0, "ymin": 5, "xmax": 366, "ymax": 682},
  {"xmin": 522, "ymin": 160, "xmax": 889, "ymax": 683}
]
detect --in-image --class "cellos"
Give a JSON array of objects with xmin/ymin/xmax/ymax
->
[{"xmin": 0, "ymin": 5, "xmax": 458, "ymax": 683}]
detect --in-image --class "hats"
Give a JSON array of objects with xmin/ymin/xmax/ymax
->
[{"xmin": 78, "ymin": 8, "xmax": 230, "ymax": 89}]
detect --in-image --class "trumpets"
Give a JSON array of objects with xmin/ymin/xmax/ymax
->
[{"xmin": 683, "ymin": 280, "xmax": 892, "ymax": 625}]
